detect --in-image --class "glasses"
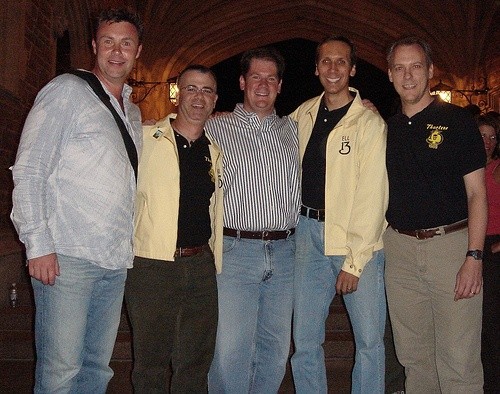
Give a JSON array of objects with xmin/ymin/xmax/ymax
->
[{"xmin": 180, "ymin": 85, "xmax": 216, "ymax": 95}]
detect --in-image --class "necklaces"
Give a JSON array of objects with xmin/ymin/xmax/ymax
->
[{"xmin": 172, "ymin": 122, "xmax": 200, "ymax": 145}]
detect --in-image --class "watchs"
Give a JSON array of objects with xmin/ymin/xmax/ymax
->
[{"xmin": 465, "ymin": 249, "xmax": 483, "ymax": 260}]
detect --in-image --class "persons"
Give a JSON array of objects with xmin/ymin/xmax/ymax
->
[
  {"xmin": 286, "ymin": 39, "xmax": 388, "ymax": 394},
  {"xmin": 9, "ymin": 6, "xmax": 144, "ymax": 394},
  {"xmin": 143, "ymin": 49, "xmax": 378, "ymax": 394},
  {"xmin": 381, "ymin": 38, "xmax": 488, "ymax": 394},
  {"xmin": 125, "ymin": 64, "xmax": 225, "ymax": 394},
  {"xmin": 463, "ymin": 103, "xmax": 500, "ymax": 394}
]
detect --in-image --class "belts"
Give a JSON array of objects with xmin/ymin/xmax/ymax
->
[
  {"xmin": 173, "ymin": 243, "xmax": 208, "ymax": 258},
  {"xmin": 300, "ymin": 205, "xmax": 328, "ymax": 222},
  {"xmin": 391, "ymin": 219, "xmax": 470, "ymax": 241},
  {"xmin": 223, "ymin": 227, "xmax": 295, "ymax": 242}
]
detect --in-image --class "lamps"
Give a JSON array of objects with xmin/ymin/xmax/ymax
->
[
  {"xmin": 128, "ymin": 74, "xmax": 180, "ymax": 106},
  {"xmin": 428, "ymin": 77, "xmax": 486, "ymax": 105}
]
